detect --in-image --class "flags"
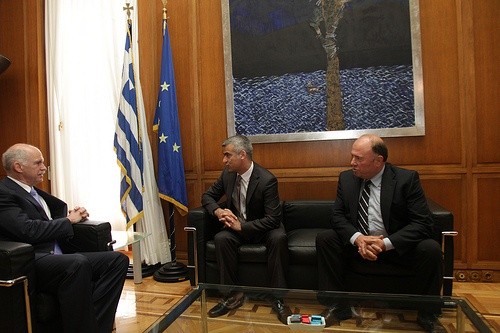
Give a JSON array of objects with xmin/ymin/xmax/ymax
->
[
  {"xmin": 153, "ymin": 19, "xmax": 189, "ymax": 217},
  {"xmin": 113, "ymin": 19, "xmax": 145, "ymax": 231}
]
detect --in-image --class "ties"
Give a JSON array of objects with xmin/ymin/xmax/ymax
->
[
  {"xmin": 359, "ymin": 181, "xmax": 372, "ymax": 236},
  {"xmin": 231, "ymin": 175, "xmax": 242, "ymax": 221},
  {"xmin": 30, "ymin": 189, "xmax": 63, "ymax": 255}
]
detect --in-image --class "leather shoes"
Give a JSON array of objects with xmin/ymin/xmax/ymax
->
[
  {"xmin": 272, "ymin": 299, "xmax": 292, "ymax": 325},
  {"xmin": 208, "ymin": 291, "xmax": 246, "ymax": 318},
  {"xmin": 319, "ymin": 303, "xmax": 352, "ymax": 327},
  {"xmin": 417, "ymin": 313, "xmax": 448, "ymax": 333}
]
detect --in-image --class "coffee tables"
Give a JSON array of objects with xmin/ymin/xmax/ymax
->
[
  {"xmin": 111, "ymin": 230, "xmax": 152, "ymax": 283},
  {"xmin": 143, "ymin": 282, "xmax": 500, "ymax": 333}
]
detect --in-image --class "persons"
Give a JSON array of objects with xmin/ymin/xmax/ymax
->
[
  {"xmin": 0, "ymin": 143, "xmax": 130, "ymax": 333},
  {"xmin": 201, "ymin": 135, "xmax": 293, "ymax": 325},
  {"xmin": 315, "ymin": 134, "xmax": 445, "ymax": 333}
]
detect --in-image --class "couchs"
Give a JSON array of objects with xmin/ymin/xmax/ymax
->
[
  {"xmin": 0, "ymin": 220, "xmax": 116, "ymax": 333},
  {"xmin": 187, "ymin": 200, "xmax": 454, "ymax": 310}
]
